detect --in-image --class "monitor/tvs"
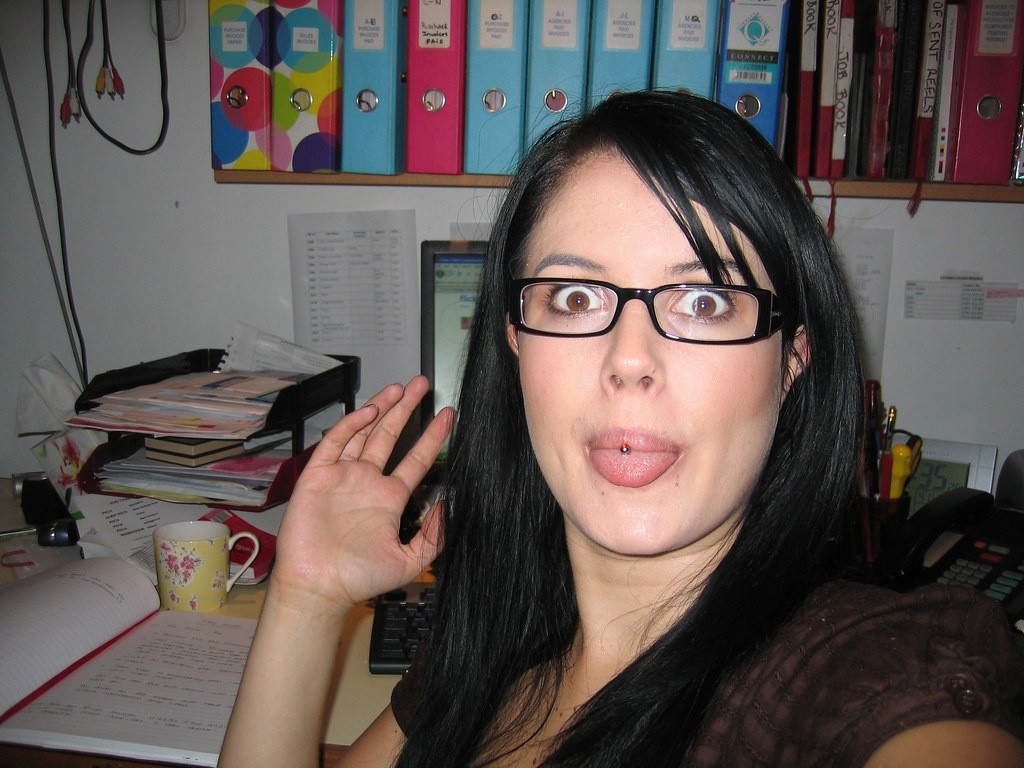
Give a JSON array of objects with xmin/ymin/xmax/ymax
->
[{"xmin": 420, "ymin": 242, "xmax": 491, "ymax": 467}]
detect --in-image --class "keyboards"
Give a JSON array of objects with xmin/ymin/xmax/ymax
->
[{"xmin": 369, "ymin": 581, "xmax": 438, "ymax": 675}]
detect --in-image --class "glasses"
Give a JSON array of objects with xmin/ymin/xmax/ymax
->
[{"xmin": 510, "ymin": 276, "xmax": 786, "ymax": 347}]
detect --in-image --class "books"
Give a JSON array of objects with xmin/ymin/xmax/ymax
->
[
  {"xmin": 795, "ymin": 0, "xmax": 1024, "ymax": 185},
  {"xmin": 0, "ymin": 368, "xmax": 317, "ymax": 768}
]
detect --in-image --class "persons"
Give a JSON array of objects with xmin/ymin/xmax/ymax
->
[{"xmin": 215, "ymin": 88, "xmax": 1024, "ymax": 768}]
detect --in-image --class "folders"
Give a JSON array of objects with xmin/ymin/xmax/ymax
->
[
  {"xmin": 203, "ymin": 0, "xmax": 792, "ymax": 178},
  {"xmin": 951, "ymin": 0, "xmax": 1024, "ymax": 184}
]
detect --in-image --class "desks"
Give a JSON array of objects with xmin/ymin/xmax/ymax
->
[{"xmin": 0, "ymin": 477, "xmax": 434, "ymax": 768}]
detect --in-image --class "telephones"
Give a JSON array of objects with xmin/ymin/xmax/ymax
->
[{"xmin": 884, "ymin": 485, "xmax": 1023, "ymax": 625}]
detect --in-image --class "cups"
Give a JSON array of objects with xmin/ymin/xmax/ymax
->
[
  {"xmin": 153, "ymin": 520, "xmax": 259, "ymax": 613},
  {"xmin": 840, "ymin": 491, "xmax": 912, "ymax": 584}
]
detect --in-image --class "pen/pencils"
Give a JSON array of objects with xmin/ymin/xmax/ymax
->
[{"xmin": 867, "ymin": 377, "xmax": 899, "ymax": 452}]
